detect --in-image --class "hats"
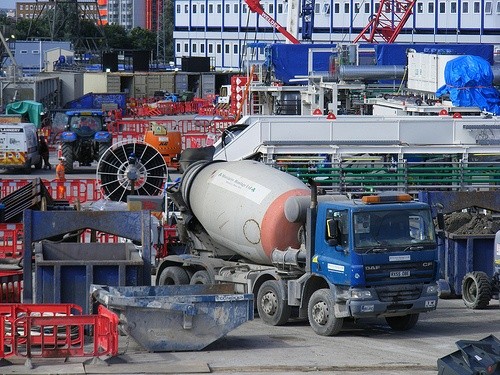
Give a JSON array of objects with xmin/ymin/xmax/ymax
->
[
  {"xmin": 40, "ymin": 136, "xmax": 44, "ymax": 138},
  {"xmin": 58, "ymin": 156, "xmax": 66, "ymax": 161}
]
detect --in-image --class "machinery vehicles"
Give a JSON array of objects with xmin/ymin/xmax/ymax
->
[
  {"xmin": 154, "ymin": 145, "xmax": 440, "ymax": 337},
  {"xmin": 60, "ymin": 110, "xmax": 112, "ymax": 174}
]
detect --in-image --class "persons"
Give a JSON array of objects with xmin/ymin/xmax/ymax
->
[{"xmin": 56, "ymin": 156, "xmax": 66, "ymax": 179}]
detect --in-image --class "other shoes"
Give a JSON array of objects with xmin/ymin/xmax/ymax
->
[
  {"xmin": 44, "ymin": 165, "xmax": 47, "ymax": 170},
  {"xmin": 49, "ymin": 164, "xmax": 51, "ymax": 170}
]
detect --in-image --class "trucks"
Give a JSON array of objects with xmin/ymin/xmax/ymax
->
[
  {"xmin": 418, "ymin": 189, "xmax": 500, "ymax": 309},
  {"xmin": 0, "ymin": 123, "xmax": 43, "ymax": 175}
]
